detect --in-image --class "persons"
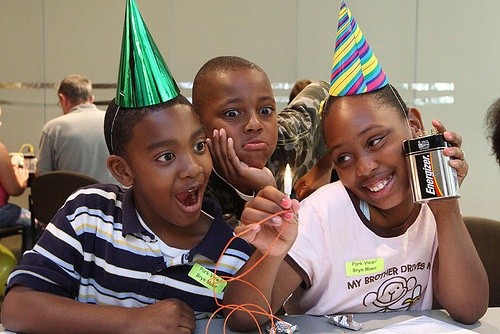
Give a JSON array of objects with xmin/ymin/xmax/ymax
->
[
  {"xmin": 223, "ymin": 1, "xmax": 490, "ymax": 332},
  {"xmin": 192, "ymin": 55, "xmax": 339, "ymax": 231},
  {"xmin": 0, "ymin": 106, "xmax": 30, "ymax": 213},
  {"xmin": 0, "ymin": 94, "xmax": 253, "ymax": 334},
  {"xmin": 0, "ymin": 0, "xmax": 254, "ymax": 334},
  {"xmin": 36, "ymin": 74, "xmax": 129, "ymax": 223}
]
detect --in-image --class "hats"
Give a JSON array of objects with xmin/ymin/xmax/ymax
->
[
  {"xmin": 116, "ymin": 0, "xmax": 180, "ymax": 107},
  {"xmin": 328, "ymin": 0, "xmax": 389, "ymax": 96}
]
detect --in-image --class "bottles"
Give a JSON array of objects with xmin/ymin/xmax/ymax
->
[{"xmin": 24, "ymin": 155, "xmax": 36, "ymax": 173}]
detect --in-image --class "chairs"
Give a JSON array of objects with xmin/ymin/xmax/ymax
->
[
  {"xmin": 28, "ymin": 170, "xmax": 102, "ymax": 250},
  {"xmin": 0, "ymin": 225, "xmax": 28, "ymax": 261},
  {"xmin": 432, "ymin": 216, "xmax": 500, "ymax": 309}
]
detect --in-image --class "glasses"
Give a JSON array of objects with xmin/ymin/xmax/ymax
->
[{"xmin": 56, "ymin": 99, "xmax": 62, "ymax": 107}]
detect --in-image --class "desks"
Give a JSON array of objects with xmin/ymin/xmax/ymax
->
[
  {"xmin": 0, "ymin": 307, "xmax": 500, "ymax": 334},
  {"xmin": 10, "ymin": 156, "xmax": 38, "ymax": 186}
]
